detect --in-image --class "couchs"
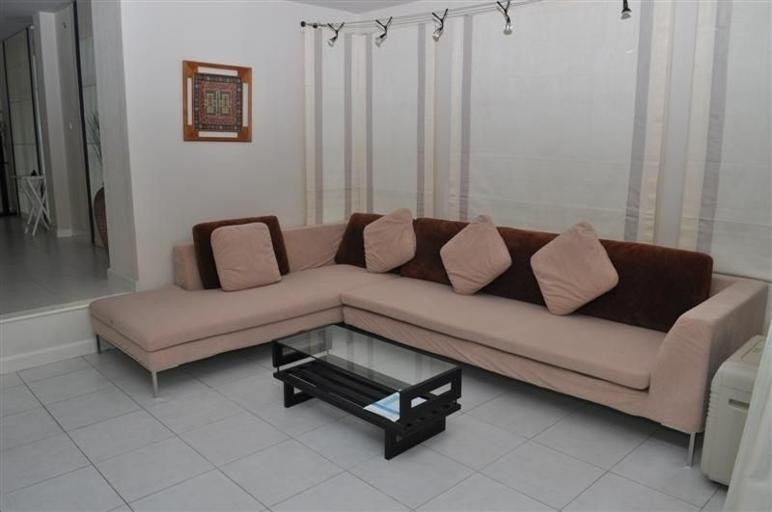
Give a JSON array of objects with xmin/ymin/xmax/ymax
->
[{"xmin": 88, "ymin": 214, "xmax": 764, "ymax": 471}]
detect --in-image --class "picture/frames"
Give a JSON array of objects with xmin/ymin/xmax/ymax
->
[{"xmin": 183, "ymin": 60, "xmax": 253, "ymax": 143}]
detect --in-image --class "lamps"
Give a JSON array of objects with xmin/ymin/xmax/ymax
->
[
  {"xmin": 495, "ymin": 0, "xmax": 515, "ymax": 36},
  {"xmin": 431, "ymin": 8, "xmax": 449, "ymax": 38},
  {"xmin": 621, "ymin": 0, "xmax": 632, "ymax": 15},
  {"xmin": 327, "ymin": 22, "xmax": 345, "ymax": 46},
  {"xmin": 373, "ymin": 16, "xmax": 393, "ymax": 43}
]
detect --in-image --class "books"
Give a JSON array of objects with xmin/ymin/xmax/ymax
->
[{"xmin": 363, "ymin": 387, "xmax": 429, "ymax": 424}]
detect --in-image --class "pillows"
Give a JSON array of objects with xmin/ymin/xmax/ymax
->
[
  {"xmin": 209, "ymin": 222, "xmax": 281, "ymax": 291},
  {"xmin": 438, "ymin": 214, "xmax": 514, "ymax": 296},
  {"xmin": 529, "ymin": 221, "xmax": 620, "ymax": 315},
  {"xmin": 363, "ymin": 208, "xmax": 417, "ymax": 274}
]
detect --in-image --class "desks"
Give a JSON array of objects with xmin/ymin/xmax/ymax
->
[{"xmin": 9, "ymin": 174, "xmax": 50, "ymax": 237}]
